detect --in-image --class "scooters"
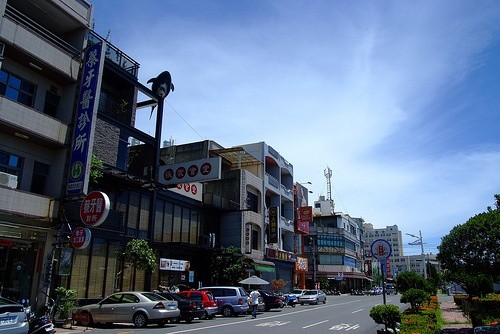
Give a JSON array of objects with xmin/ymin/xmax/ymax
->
[{"xmin": 24, "ymin": 305, "xmax": 57, "ymax": 334}]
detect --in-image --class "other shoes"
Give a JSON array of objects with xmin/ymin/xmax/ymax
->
[{"xmin": 252, "ymin": 316, "xmax": 256, "ymax": 319}]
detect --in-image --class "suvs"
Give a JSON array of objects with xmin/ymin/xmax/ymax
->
[{"xmin": 196, "ymin": 286, "xmax": 250, "ymax": 318}]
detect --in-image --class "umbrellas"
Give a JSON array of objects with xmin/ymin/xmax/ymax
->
[{"xmin": 238, "ymin": 276, "xmax": 270, "ymax": 284}]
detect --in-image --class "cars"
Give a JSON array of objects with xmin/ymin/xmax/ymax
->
[
  {"xmin": 298, "ymin": 289, "xmax": 327, "ymax": 305},
  {"xmin": 156, "ymin": 292, "xmax": 197, "ymax": 323},
  {"xmin": 71, "ymin": 291, "xmax": 180, "ymax": 328},
  {"xmin": 0, "ymin": 296, "xmax": 30, "ymax": 334},
  {"xmin": 287, "ymin": 289, "xmax": 311, "ymax": 304},
  {"xmin": 175, "ymin": 289, "xmax": 219, "ymax": 321},
  {"xmin": 369, "ymin": 279, "xmax": 398, "ymax": 296},
  {"xmin": 246, "ymin": 288, "xmax": 297, "ymax": 314}
]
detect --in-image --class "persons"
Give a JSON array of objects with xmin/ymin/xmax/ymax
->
[
  {"xmin": 270, "ymin": 288, "xmax": 284, "ymax": 308},
  {"xmin": 249, "ymin": 286, "xmax": 261, "ymax": 319}
]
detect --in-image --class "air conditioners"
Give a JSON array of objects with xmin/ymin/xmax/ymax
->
[{"xmin": 0, "ymin": 172, "xmax": 17, "ymax": 189}]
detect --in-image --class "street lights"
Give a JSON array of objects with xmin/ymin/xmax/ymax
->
[{"xmin": 405, "ymin": 229, "xmax": 427, "ymax": 280}]
